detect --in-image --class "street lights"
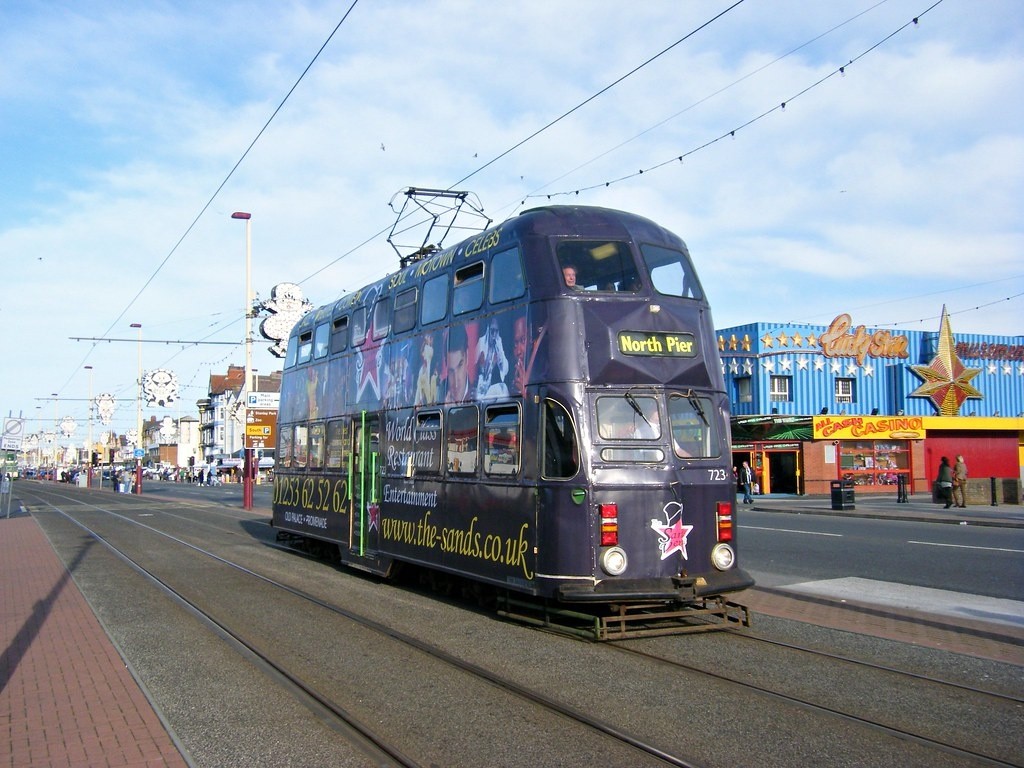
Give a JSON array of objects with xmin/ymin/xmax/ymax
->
[
  {"xmin": 51, "ymin": 393, "xmax": 59, "ymax": 483},
  {"xmin": 83, "ymin": 365, "xmax": 94, "ymax": 489},
  {"xmin": 35, "ymin": 406, "xmax": 43, "ymax": 480},
  {"xmin": 229, "ymin": 212, "xmax": 260, "ymax": 512},
  {"xmin": 130, "ymin": 323, "xmax": 143, "ymax": 495}
]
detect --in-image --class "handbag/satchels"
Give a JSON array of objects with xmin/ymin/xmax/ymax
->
[
  {"xmin": 753, "ymin": 483, "xmax": 760, "ymax": 493},
  {"xmin": 936, "ymin": 483, "xmax": 945, "ymax": 499},
  {"xmin": 953, "ymin": 472, "xmax": 959, "ymax": 487}
]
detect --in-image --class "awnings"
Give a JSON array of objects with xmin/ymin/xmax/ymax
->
[
  {"xmin": 217, "ymin": 463, "xmax": 240, "ymax": 468},
  {"xmin": 253, "ymin": 464, "xmax": 274, "ymax": 468}
]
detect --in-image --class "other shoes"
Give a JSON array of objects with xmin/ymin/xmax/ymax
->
[
  {"xmin": 944, "ymin": 503, "xmax": 950, "ymax": 508},
  {"xmin": 743, "ymin": 499, "xmax": 748, "ymax": 504},
  {"xmin": 960, "ymin": 505, "xmax": 966, "ymax": 508},
  {"xmin": 953, "ymin": 505, "xmax": 959, "ymax": 508},
  {"xmin": 749, "ymin": 499, "xmax": 754, "ymax": 504}
]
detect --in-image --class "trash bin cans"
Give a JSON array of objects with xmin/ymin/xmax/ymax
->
[
  {"xmin": 76, "ymin": 474, "xmax": 87, "ymax": 488},
  {"xmin": 830, "ymin": 480, "xmax": 856, "ymax": 511},
  {"xmin": 932, "ymin": 481, "xmax": 946, "ymax": 503}
]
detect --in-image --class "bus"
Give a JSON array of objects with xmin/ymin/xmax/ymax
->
[{"xmin": 268, "ymin": 185, "xmax": 758, "ymax": 645}]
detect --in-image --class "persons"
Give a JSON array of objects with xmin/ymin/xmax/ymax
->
[
  {"xmin": 936, "ymin": 457, "xmax": 953, "ymax": 509},
  {"xmin": 159, "ymin": 467, "xmax": 170, "ymax": 482},
  {"xmin": 732, "ymin": 467, "xmax": 738, "ymax": 480},
  {"xmin": 601, "ymin": 279, "xmax": 616, "ymax": 291},
  {"xmin": 26, "ymin": 471, "xmax": 33, "ymax": 479},
  {"xmin": 563, "ymin": 265, "xmax": 584, "ymax": 291},
  {"xmin": 113, "ymin": 468, "xmax": 132, "ymax": 494},
  {"xmin": 952, "ymin": 455, "xmax": 968, "ymax": 508},
  {"xmin": 146, "ymin": 471, "xmax": 153, "ymax": 480},
  {"xmin": 384, "ymin": 318, "xmax": 545, "ymax": 405},
  {"xmin": 61, "ymin": 471, "xmax": 66, "ymax": 482},
  {"xmin": 740, "ymin": 461, "xmax": 756, "ymax": 504},
  {"xmin": 40, "ymin": 471, "xmax": 44, "ymax": 481},
  {"xmin": 634, "ymin": 407, "xmax": 692, "ymax": 460},
  {"xmin": 179, "ymin": 468, "xmax": 213, "ymax": 487},
  {"xmin": 621, "ymin": 280, "xmax": 639, "ymax": 290}
]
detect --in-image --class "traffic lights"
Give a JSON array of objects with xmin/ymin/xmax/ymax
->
[
  {"xmin": 109, "ymin": 449, "xmax": 115, "ymax": 463},
  {"xmin": 206, "ymin": 456, "xmax": 210, "ymax": 464}
]
